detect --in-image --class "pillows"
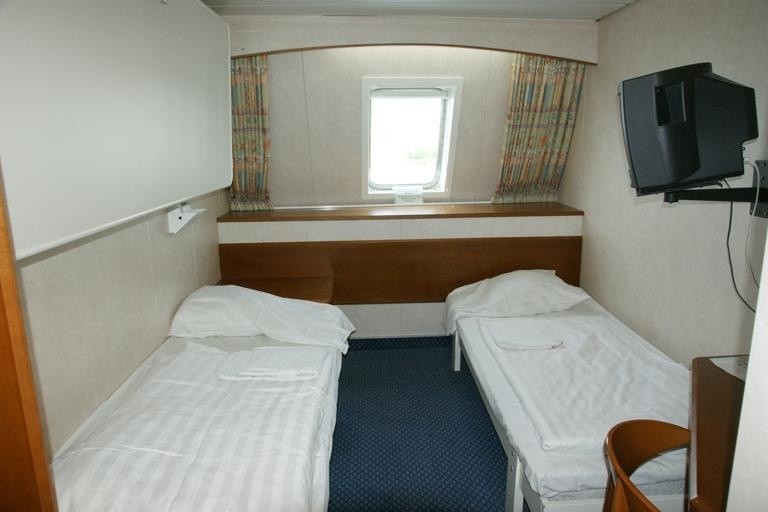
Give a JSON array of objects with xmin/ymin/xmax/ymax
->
[
  {"xmin": 440, "ymin": 266, "xmax": 592, "ymax": 337},
  {"xmin": 171, "ymin": 279, "xmax": 359, "ymax": 355}
]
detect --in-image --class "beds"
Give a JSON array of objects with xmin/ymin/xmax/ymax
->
[
  {"xmin": 42, "ymin": 324, "xmax": 340, "ymax": 512},
  {"xmin": 450, "ymin": 306, "xmax": 694, "ymax": 511}
]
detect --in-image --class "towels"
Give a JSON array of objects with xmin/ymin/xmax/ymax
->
[
  {"xmin": 488, "ymin": 318, "xmax": 567, "ymax": 351},
  {"xmin": 216, "ymin": 344, "xmax": 327, "ymax": 382}
]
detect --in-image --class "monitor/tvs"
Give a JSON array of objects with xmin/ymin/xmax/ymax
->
[{"xmin": 616, "ymin": 61, "xmax": 759, "ymax": 197}]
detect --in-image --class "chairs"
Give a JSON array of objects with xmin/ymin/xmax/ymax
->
[{"xmin": 603, "ymin": 409, "xmax": 689, "ymax": 510}]
